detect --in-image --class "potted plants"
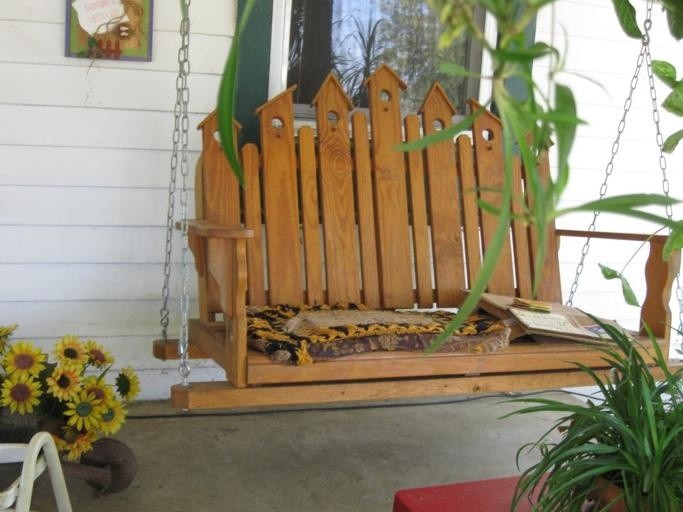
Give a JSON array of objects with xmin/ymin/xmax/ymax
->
[{"xmin": 498, "ymin": 306, "xmax": 681, "ymax": 511}]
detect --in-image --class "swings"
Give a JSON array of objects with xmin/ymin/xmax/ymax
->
[{"xmin": 153, "ymin": 1, "xmax": 682, "ymax": 409}]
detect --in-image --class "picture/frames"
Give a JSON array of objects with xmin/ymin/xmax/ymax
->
[{"xmin": 63, "ymin": 1, "xmax": 154, "ymax": 63}]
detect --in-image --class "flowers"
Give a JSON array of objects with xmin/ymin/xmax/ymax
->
[{"xmin": 0, "ymin": 323, "xmax": 141, "ymax": 461}]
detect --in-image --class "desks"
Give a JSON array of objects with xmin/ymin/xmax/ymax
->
[{"xmin": 390, "ymin": 469, "xmax": 595, "ymax": 512}]
